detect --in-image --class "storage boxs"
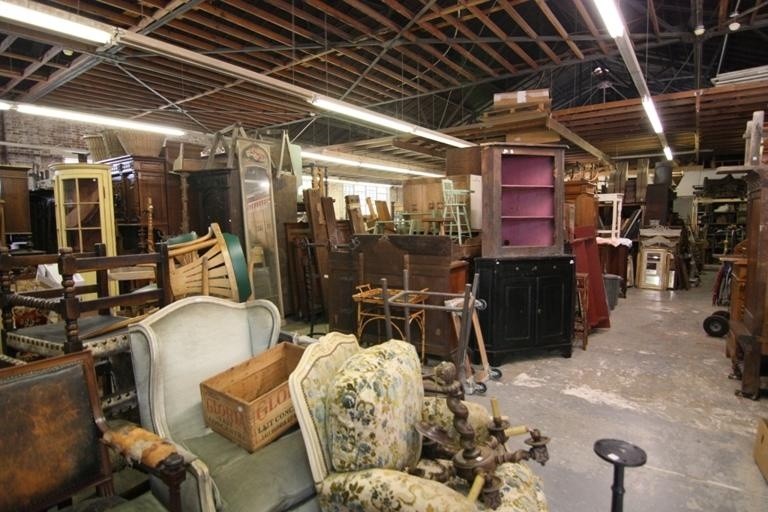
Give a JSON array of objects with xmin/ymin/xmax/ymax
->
[{"xmin": 480, "ymin": 88, "xmax": 561, "ymax": 143}]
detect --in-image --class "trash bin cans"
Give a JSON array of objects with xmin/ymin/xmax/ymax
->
[{"xmin": 602, "ymin": 274, "xmax": 624, "ymax": 310}]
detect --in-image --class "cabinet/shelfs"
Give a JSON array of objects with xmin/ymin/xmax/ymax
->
[
  {"xmin": 467, "ymin": 255, "xmax": 576, "ymax": 367},
  {"xmin": 479, "ymin": 138, "xmax": 569, "ymax": 258},
  {"xmin": 698, "ymin": 103, "xmax": 767, "ymax": 401}
]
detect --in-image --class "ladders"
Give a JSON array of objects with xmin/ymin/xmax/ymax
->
[{"xmin": 441, "ymin": 204, "xmax": 472, "ymax": 248}]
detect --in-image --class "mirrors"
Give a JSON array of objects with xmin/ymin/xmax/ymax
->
[{"xmin": 235, "ymin": 136, "xmax": 286, "ymax": 327}]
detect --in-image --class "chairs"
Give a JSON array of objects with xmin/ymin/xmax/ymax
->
[{"xmin": 438, "ymin": 177, "xmax": 472, "ymax": 246}]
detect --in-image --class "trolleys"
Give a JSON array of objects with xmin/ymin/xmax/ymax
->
[
  {"xmin": 445, "ymin": 289, "xmax": 503, "ymax": 395},
  {"xmin": 702, "ymin": 226, "xmax": 743, "ymax": 338}
]
